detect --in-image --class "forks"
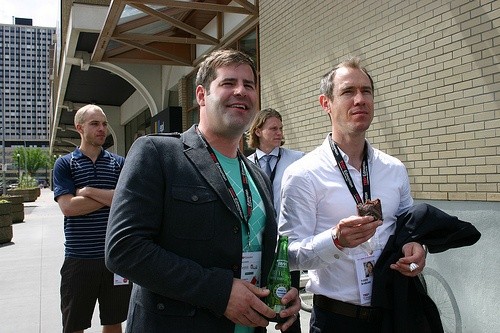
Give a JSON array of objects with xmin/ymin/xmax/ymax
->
[{"xmin": 409, "ymin": 263, "xmax": 419, "ymax": 272}]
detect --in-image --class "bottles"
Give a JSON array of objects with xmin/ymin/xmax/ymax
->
[{"xmin": 265, "ymin": 235, "xmax": 291, "ymax": 323}]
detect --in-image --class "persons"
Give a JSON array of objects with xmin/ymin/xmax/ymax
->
[
  {"xmin": 365, "ymin": 261, "xmax": 374, "ymax": 277},
  {"xmin": 105, "ymin": 47, "xmax": 302, "ymax": 333},
  {"xmin": 247, "ymin": 106, "xmax": 308, "ymax": 332},
  {"xmin": 52, "ymin": 103, "xmax": 133, "ymax": 333}
]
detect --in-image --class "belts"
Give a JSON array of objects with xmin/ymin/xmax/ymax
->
[{"xmin": 313, "ymin": 294, "xmax": 370, "ymax": 320}]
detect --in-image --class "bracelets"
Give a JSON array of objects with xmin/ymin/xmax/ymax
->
[
  {"xmin": 331, "ymin": 225, "xmax": 344, "ymax": 253},
  {"xmin": 276, "ymin": 58, "xmax": 427, "ymax": 333},
  {"xmin": 419, "ymin": 241, "xmax": 428, "ymax": 259}
]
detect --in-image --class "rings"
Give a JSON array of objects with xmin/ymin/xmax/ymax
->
[{"xmin": 293, "ymin": 314, "xmax": 299, "ymax": 319}]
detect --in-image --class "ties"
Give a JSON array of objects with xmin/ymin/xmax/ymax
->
[{"xmin": 262, "ymin": 155, "xmax": 273, "ymax": 178}]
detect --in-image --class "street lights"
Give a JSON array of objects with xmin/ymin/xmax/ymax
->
[{"xmin": 16, "ymin": 151, "xmax": 20, "ymax": 180}]
